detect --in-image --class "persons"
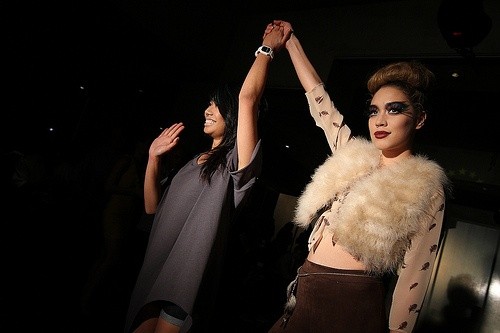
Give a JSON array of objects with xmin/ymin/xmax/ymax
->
[
  {"xmin": 262, "ymin": 19, "xmax": 455, "ymax": 332},
  {"xmin": 124, "ymin": 19, "xmax": 291, "ymax": 333}
]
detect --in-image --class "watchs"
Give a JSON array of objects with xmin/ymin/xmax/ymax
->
[{"xmin": 255, "ymin": 45, "xmax": 276, "ymax": 63}]
